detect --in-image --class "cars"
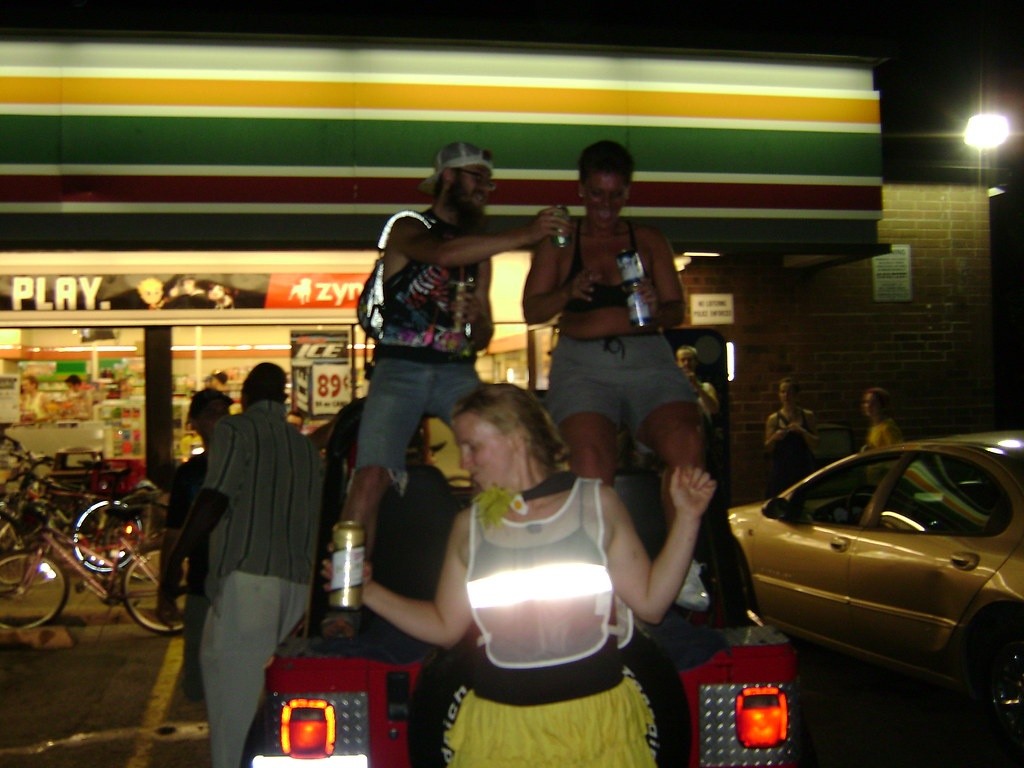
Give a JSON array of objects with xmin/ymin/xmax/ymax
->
[{"xmin": 727, "ymin": 431, "xmax": 1024, "ymax": 741}]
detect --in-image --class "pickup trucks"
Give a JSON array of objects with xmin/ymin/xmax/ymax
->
[{"xmin": 249, "ymin": 385, "xmax": 801, "ymax": 768}]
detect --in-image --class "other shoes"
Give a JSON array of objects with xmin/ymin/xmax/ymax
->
[{"xmin": 320, "ymin": 609, "xmax": 363, "ymax": 639}]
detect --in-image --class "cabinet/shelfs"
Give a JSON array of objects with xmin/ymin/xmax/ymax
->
[{"xmin": 30, "ymin": 373, "xmax": 248, "ymax": 463}]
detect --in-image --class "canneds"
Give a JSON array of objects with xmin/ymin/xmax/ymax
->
[
  {"xmin": 551, "ymin": 205, "xmax": 571, "ymax": 247},
  {"xmin": 327, "ymin": 521, "xmax": 365, "ymax": 611},
  {"xmin": 616, "ymin": 249, "xmax": 655, "ymax": 327}
]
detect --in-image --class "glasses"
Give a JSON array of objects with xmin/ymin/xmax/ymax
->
[{"xmin": 450, "ymin": 166, "xmax": 495, "ymax": 192}]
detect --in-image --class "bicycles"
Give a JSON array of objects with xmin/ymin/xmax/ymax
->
[
  {"xmin": 0, "ymin": 501, "xmax": 187, "ymax": 636},
  {"xmin": 0, "ymin": 434, "xmax": 167, "ymax": 574}
]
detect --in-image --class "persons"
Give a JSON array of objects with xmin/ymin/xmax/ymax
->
[
  {"xmin": 164, "ymin": 362, "xmax": 325, "ymax": 768},
  {"xmin": 321, "ymin": 141, "xmax": 574, "ymax": 638},
  {"xmin": 320, "ymin": 385, "xmax": 716, "ymax": 768},
  {"xmin": 676, "ymin": 347, "xmax": 719, "ymax": 477},
  {"xmin": 156, "ymin": 388, "xmax": 234, "ymax": 699},
  {"xmin": 523, "ymin": 139, "xmax": 710, "ymax": 609},
  {"xmin": 859, "ymin": 388, "xmax": 903, "ymax": 484},
  {"xmin": 764, "ymin": 378, "xmax": 820, "ymax": 498},
  {"xmin": 65, "ymin": 375, "xmax": 96, "ymax": 394},
  {"xmin": 20, "ymin": 376, "xmax": 53, "ymax": 423},
  {"xmin": 212, "ymin": 369, "xmax": 229, "ymax": 395}
]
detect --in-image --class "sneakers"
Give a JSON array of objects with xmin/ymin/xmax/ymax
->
[{"xmin": 676, "ymin": 557, "xmax": 712, "ymax": 612}]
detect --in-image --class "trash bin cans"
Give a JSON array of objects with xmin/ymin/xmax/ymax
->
[{"xmin": 810, "ymin": 424, "xmax": 857, "ymax": 467}]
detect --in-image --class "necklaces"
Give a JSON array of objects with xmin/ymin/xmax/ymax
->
[{"xmin": 470, "ymin": 470, "xmax": 576, "ymax": 530}]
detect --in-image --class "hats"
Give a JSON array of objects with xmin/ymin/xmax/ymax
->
[
  {"xmin": 189, "ymin": 389, "xmax": 233, "ymax": 419},
  {"xmin": 418, "ymin": 142, "xmax": 493, "ymax": 195}
]
